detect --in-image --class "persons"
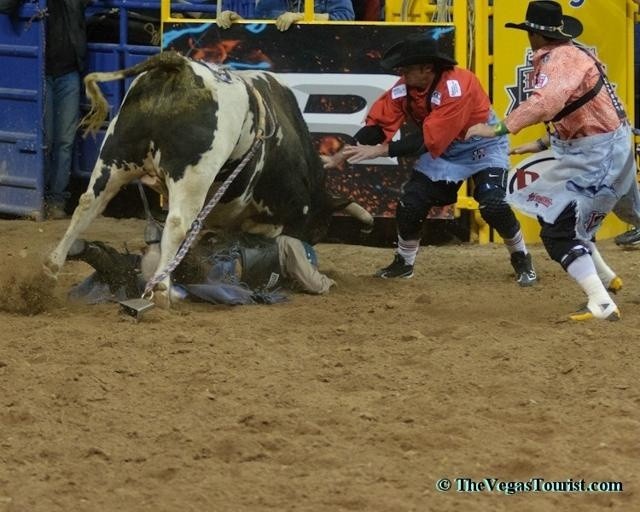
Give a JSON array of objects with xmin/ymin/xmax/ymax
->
[
  {"xmin": 137, "ymin": 0, "xmax": 216, "ymax": 47},
  {"xmin": 608, "ymin": 173, "xmax": 640, "ymax": 249},
  {"xmin": 461, "ymin": 1, "xmax": 637, "ymax": 326},
  {"xmin": 314, "ymin": 31, "xmax": 538, "ymax": 289},
  {"xmin": 214, "ymin": 0, "xmax": 358, "ymax": 34},
  {"xmin": 40, "ymin": 1, "xmax": 89, "ymax": 221},
  {"xmin": 311, "ymin": 136, "xmax": 405, "ymax": 245},
  {"xmin": 63, "ymin": 227, "xmax": 338, "ymax": 305}
]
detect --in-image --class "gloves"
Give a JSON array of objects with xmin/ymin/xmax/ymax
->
[
  {"xmin": 275, "ymin": 12, "xmax": 331, "ymax": 33},
  {"xmin": 215, "ymin": 10, "xmax": 243, "ymax": 30}
]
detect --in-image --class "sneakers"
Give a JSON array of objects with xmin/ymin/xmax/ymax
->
[
  {"xmin": 46, "ymin": 206, "xmax": 69, "ymax": 221},
  {"xmin": 615, "ymin": 226, "xmax": 640, "ymax": 246},
  {"xmin": 509, "ymin": 251, "xmax": 537, "ymax": 287},
  {"xmin": 376, "ymin": 253, "xmax": 414, "ymax": 279},
  {"xmin": 568, "ymin": 297, "xmax": 623, "ymax": 322},
  {"xmin": 606, "ymin": 274, "xmax": 625, "ymax": 295},
  {"xmin": 140, "ymin": 222, "xmax": 163, "ymax": 284},
  {"xmin": 63, "ymin": 237, "xmax": 90, "ymax": 261}
]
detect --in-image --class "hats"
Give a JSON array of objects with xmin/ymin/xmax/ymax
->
[
  {"xmin": 504, "ymin": 0, "xmax": 588, "ymax": 41},
  {"xmin": 381, "ymin": 32, "xmax": 460, "ymax": 73}
]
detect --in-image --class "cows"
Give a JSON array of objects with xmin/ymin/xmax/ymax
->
[{"xmin": 41, "ymin": 50, "xmax": 374, "ymax": 311}]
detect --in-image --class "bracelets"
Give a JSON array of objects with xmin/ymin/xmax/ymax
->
[
  {"xmin": 536, "ymin": 138, "xmax": 549, "ymax": 152},
  {"xmin": 492, "ymin": 122, "xmax": 512, "ymax": 137}
]
